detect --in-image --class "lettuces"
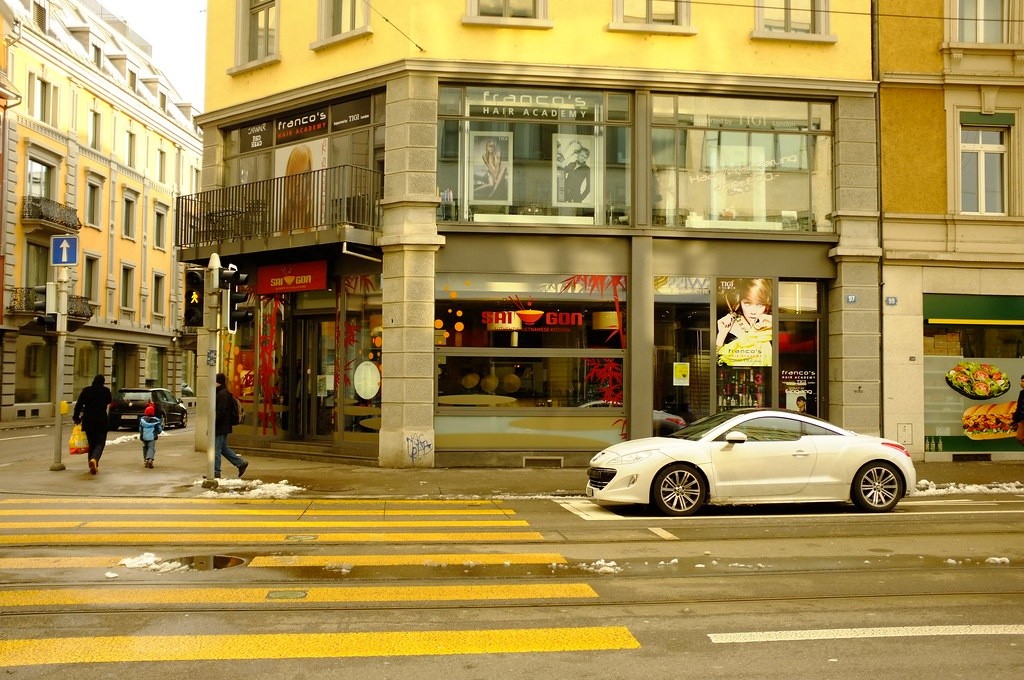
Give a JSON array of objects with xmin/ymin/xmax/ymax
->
[{"xmin": 945, "ymin": 361, "xmax": 1009, "ymax": 396}]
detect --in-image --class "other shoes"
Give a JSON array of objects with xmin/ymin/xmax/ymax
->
[
  {"xmin": 89, "ymin": 459, "xmax": 98, "ymax": 474},
  {"xmin": 238, "ymin": 461, "xmax": 248, "ymax": 477},
  {"xmin": 144, "ymin": 458, "xmax": 154, "ymax": 468},
  {"xmin": 203, "ymin": 473, "xmax": 221, "ymax": 479}
]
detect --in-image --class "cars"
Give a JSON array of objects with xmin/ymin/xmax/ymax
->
[
  {"xmin": 108, "ymin": 387, "xmax": 188, "ymax": 431},
  {"xmin": 181, "ymin": 383, "xmax": 195, "ymax": 396}
]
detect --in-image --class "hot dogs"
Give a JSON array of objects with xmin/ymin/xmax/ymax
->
[{"xmin": 962, "ymin": 401, "xmax": 1019, "ymax": 440}]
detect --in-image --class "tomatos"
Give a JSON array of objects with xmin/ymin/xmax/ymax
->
[
  {"xmin": 954, "ymin": 366, "xmax": 966, "ymax": 372},
  {"xmin": 974, "ymin": 383, "xmax": 989, "ymax": 394},
  {"xmin": 981, "ymin": 363, "xmax": 992, "ymax": 371},
  {"xmin": 992, "ymin": 372, "xmax": 1001, "ymax": 380},
  {"xmin": 956, "ymin": 374, "xmax": 971, "ymax": 382},
  {"xmin": 973, "ymin": 370, "xmax": 988, "ymax": 380}
]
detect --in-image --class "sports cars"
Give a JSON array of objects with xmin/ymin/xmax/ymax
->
[
  {"xmin": 576, "ymin": 398, "xmax": 687, "ymax": 438},
  {"xmin": 585, "ymin": 406, "xmax": 917, "ymax": 516}
]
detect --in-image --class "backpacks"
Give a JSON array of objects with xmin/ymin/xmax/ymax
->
[{"xmin": 230, "ymin": 393, "xmax": 245, "ymax": 426}]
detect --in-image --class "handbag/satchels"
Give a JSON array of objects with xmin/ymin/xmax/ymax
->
[{"xmin": 68, "ymin": 422, "xmax": 89, "ymax": 455}]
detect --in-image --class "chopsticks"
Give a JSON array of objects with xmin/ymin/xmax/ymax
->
[{"xmin": 508, "ymin": 295, "xmax": 525, "ymax": 310}]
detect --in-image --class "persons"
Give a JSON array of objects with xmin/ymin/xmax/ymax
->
[
  {"xmin": 714, "ymin": 277, "xmax": 774, "ymax": 365},
  {"xmin": 72, "ymin": 374, "xmax": 112, "ymax": 474},
  {"xmin": 563, "ymin": 148, "xmax": 592, "ymax": 203},
  {"xmin": 473, "ymin": 140, "xmax": 506, "ymax": 199},
  {"xmin": 1011, "ymin": 375, "xmax": 1024, "ymax": 447},
  {"xmin": 797, "ymin": 396, "xmax": 806, "ymax": 413},
  {"xmin": 279, "ymin": 144, "xmax": 315, "ymax": 228},
  {"xmin": 139, "ymin": 402, "xmax": 162, "ymax": 468},
  {"xmin": 214, "ymin": 373, "xmax": 248, "ymax": 478}
]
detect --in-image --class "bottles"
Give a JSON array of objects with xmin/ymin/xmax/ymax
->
[
  {"xmin": 938, "ymin": 437, "xmax": 942, "ymax": 452},
  {"xmin": 931, "ymin": 437, "xmax": 935, "ymax": 452},
  {"xmin": 925, "ymin": 437, "xmax": 929, "ymax": 452},
  {"xmin": 718, "ymin": 370, "xmax": 761, "ymax": 414}
]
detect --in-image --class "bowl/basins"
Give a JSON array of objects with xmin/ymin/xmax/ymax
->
[
  {"xmin": 516, "ymin": 310, "xmax": 544, "ymax": 325},
  {"xmin": 945, "ymin": 370, "xmax": 1010, "ymax": 400}
]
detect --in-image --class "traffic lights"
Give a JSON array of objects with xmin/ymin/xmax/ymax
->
[
  {"xmin": 228, "ymin": 263, "xmax": 254, "ymax": 335},
  {"xmin": 36, "ymin": 312, "xmax": 59, "ymax": 332},
  {"xmin": 218, "ymin": 267, "xmax": 241, "ymax": 290},
  {"xmin": 32, "ymin": 283, "xmax": 46, "ymax": 326},
  {"xmin": 183, "ymin": 267, "xmax": 206, "ymax": 329}
]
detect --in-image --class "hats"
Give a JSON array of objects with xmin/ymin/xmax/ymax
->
[
  {"xmin": 216, "ymin": 373, "xmax": 226, "ymax": 384},
  {"xmin": 144, "ymin": 402, "xmax": 155, "ymax": 416}
]
